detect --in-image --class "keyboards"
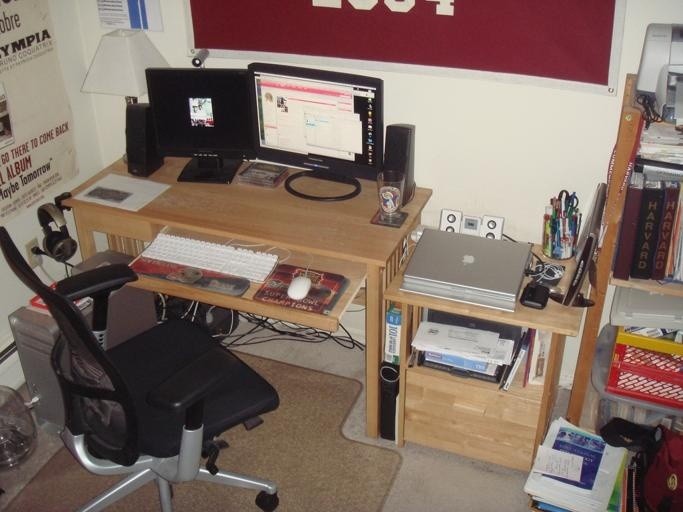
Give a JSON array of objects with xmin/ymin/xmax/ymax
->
[{"xmin": 141, "ymin": 233, "xmax": 280, "ymax": 283}]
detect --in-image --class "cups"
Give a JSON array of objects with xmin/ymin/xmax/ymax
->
[{"xmin": 377, "ymin": 170, "xmax": 404, "ymax": 219}]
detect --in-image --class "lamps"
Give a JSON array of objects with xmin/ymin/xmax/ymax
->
[{"xmin": 81, "ymin": 29, "xmax": 169, "ymax": 163}]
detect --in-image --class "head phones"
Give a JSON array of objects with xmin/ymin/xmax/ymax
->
[{"xmin": 38, "ymin": 203, "xmax": 77, "ymax": 263}]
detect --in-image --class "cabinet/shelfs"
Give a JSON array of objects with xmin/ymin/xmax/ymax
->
[{"xmin": 564, "ymin": 73, "xmax": 683, "ymax": 426}]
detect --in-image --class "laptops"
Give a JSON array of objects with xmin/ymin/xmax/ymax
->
[{"xmin": 403, "ymin": 228, "xmax": 530, "ymax": 301}]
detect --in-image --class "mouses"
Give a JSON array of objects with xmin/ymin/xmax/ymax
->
[{"xmin": 287, "ymin": 276, "xmax": 311, "ymax": 301}]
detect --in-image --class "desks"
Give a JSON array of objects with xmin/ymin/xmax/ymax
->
[
  {"xmin": 383, "ymin": 245, "xmax": 589, "ymax": 472},
  {"xmin": 61, "ymin": 155, "xmax": 432, "ymax": 439}
]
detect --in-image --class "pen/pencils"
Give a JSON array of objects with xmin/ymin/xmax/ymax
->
[{"xmin": 544, "ymin": 191, "xmax": 580, "ymax": 259}]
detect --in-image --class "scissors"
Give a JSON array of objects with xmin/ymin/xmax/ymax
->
[{"xmin": 558, "ymin": 189, "xmax": 578, "ymax": 218}]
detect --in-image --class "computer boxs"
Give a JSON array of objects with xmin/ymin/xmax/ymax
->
[{"xmin": 7, "ymin": 249, "xmax": 158, "ymax": 434}]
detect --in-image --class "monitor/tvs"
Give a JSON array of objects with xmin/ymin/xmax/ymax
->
[
  {"xmin": 146, "ymin": 67, "xmax": 256, "ymax": 185},
  {"xmin": 550, "ymin": 183, "xmax": 607, "ymax": 308},
  {"xmin": 248, "ymin": 62, "xmax": 384, "ymax": 201}
]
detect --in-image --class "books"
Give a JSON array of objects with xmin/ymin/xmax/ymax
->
[
  {"xmin": 25, "ymin": 281, "xmax": 89, "ymax": 319},
  {"xmin": 600, "ymin": 122, "xmax": 680, "ymax": 289},
  {"xmin": 236, "ymin": 160, "xmax": 289, "ymax": 184},
  {"xmin": 237, "ymin": 171, "xmax": 289, "ymax": 189},
  {"xmin": 380, "ymin": 298, "xmax": 552, "ymax": 399},
  {"xmin": 521, "ymin": 395, "xmax": 682, "ymax": 512},
  {"xmin": 69, "ymin": 232, "xmax": 151, "ymax": 275}
]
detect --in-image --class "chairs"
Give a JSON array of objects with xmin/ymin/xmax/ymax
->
[{"xmin": 0, "ymin": 226, "xmax": 279, "ymax": 512}]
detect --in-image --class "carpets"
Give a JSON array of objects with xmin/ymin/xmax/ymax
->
[{"xmin": 0, "ymin": 350, "xmax": 402, "ymax": 512}]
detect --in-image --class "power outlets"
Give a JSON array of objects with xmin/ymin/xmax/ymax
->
[{"xmin": 26, "ymin": 237, "xmax": 44, "ymax": 269}]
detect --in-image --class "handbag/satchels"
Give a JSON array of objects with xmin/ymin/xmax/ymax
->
[{"xmin": 602, "ymin": 417, "xmax": 683, "ymax": 512}]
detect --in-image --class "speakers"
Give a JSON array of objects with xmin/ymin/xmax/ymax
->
[
  {"xmin": 440, "ymin": 209, "xmax": 504, "ymax": 241},
  {"xmin": 126, "ymin": 103, "xmax": 163, "ymax": 179},
  {"xmin": 385, "ymin": 124, "xmax": 415, "ymax": 206}
]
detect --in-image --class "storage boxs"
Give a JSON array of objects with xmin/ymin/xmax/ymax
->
[{"xmin": 590, "ymin": 323, "xmax": 683, "ymax": 436}]
